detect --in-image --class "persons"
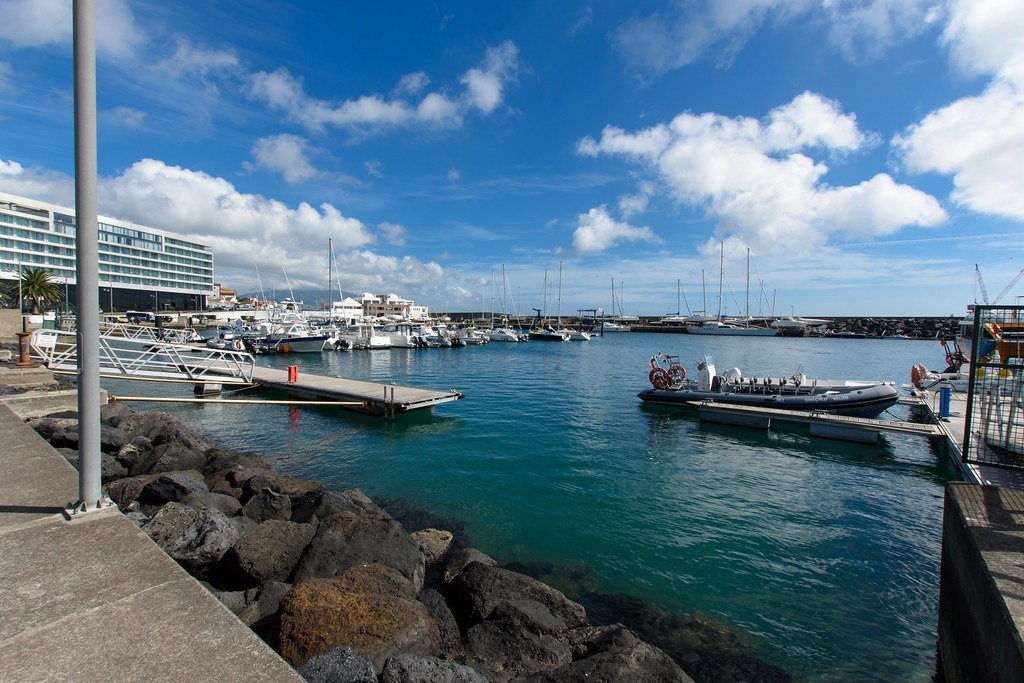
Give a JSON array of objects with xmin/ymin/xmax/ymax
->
[
  {"xmin": 135, "ymin": 316, "xmax": 140, "ymax": 324},
  {"xmin": 129, "ymin": 316, "xmax": 134, "ymax": 325},
  {"xmin": 113, "ymin": 317, "xmax": 119, "ymax": 322}
]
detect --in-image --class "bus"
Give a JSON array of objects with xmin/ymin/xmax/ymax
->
[{"xmin": 103, "ymin": 310, "xmax": 174, "ymax": 323}]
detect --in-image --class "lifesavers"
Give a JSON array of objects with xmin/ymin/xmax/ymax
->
[
  {"xmin": 236, "ymin": 341, "xmax": 241, "ymax": 348},
  {"xmin": 669, "ymin": 365, "xmax": 686, "ymax": 381},
  {"xmin": 649, "ymin": 368, "xmax": 669, "ymax": 387}
]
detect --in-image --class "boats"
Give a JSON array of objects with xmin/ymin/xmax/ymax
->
[
  {"xmin": 636, "ymin": 350, "xmax": 901, "ymax": 419},
  {"xmin": 141, "ymin": 325, "xmax": 215, "ymax": 343},
  {"xmin": 882, "ymin": 334, "xmax": 911, "ymax": 340}
]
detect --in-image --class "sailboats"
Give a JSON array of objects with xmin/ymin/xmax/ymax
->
[
  {"xmin": 649, "ymin": 240, "xmax": 835, "ymax": 337},
  {"xmin": 206, "ymin": 237, "xmax": 639, "ymax": 352}
]
